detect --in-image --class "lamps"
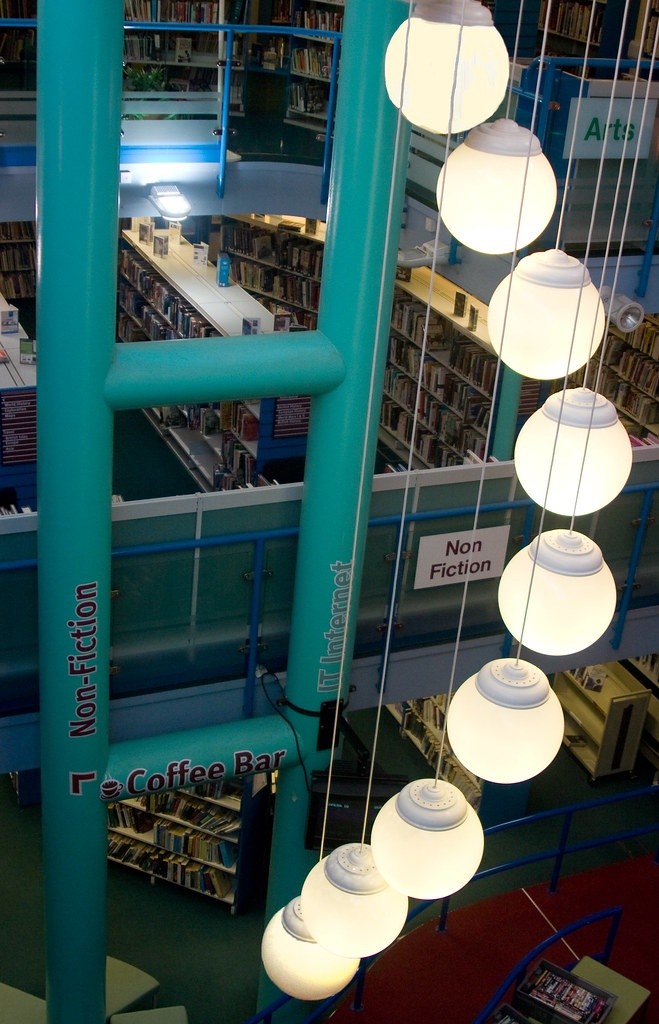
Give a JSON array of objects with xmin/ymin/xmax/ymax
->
[{"xmin": 260, "ymin": 0, "xmax": 659, "ymax": 1001}]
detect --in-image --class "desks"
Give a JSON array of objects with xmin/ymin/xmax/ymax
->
[
  {"xmin": 527, "ymin": 955, "xmax": 650, "ymax": 1024},
  {"xmin": 0, "ymin": 955, "xmax": 188, "ymax": 1024}
]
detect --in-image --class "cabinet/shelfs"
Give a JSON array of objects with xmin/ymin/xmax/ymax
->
[
  {"xmin": 0, "ymin": 212, "xmax": 659, "ymax": 494},
  {"xmin": 534, "ymin": 0, "xmax": 659, "ymax": 84},
  {"xmin": 552, "ymin": 661, "xmax": 651, "ymax": 781},
  {"xmin": 386, "ymin": 692, "xmax": 530, "ymax": 830},
  {"xmin": 123, "ymin": 0, "xmax": 343, "ymax": 114},
  {"xmin": 106, "ymin": 774, "xmax": 272, "ymax": 915}
]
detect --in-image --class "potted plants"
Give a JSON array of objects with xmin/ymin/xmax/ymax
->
[{"xmin": 125, "ymin": 62, "xmax": 170, "ymax": 120}]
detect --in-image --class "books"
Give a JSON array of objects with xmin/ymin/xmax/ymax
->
[
  {"xmin": 112, "ymin": 493, "xmax": 123, "ymax": 503},
  {"xmin": 0, "ymin": 0, "xmax": 38, "ymax": 91},
  {"xmin": 635, "ymin": 653, "xmax": 659, "ymax": 683},
  {"xmin": 1, "ymin": 304, "xmax": 19, "ymax": 333},
  {"xmin": 395, "ymin": 693, "xmax": 483, "ymax": 809},
  {"xmin": 540, "ymin": 1, "xmax": 659, "ymax": 80},
  {"xmin": 0, "ymin": 221, "xmax": 36, "ymax": 300},
  {"xmin": 124, "ymin": 1, "xmax": 345, "ymax": 113},
  {"xmin": 219, "ymin": 222, "xmax": 323, "ymax": 334},
  {"xmin": 378, "ymin": 286, "xmax": 497, "ymax": 473},
  {"xmin": 497, "ymin": 969, "xmax": 606, "ymax": 1024},
  {"xmin": 564, "ymin": 735, "xmax": 587, "ymax": 747},
  {"xmin": 570, "ymin": 665, "xmax": 607, "ymax": 692},
  {"xmin": 107, "ymin": 777, "xmax": 242, "ymax": 898},
  {"xmin": 0, "ymin": 503, "xmax": 32, "ymax": 516},
  {"xmin": 0, "ymin": 350, "xmax": 8, "ymax": 363},
  {"xmin": 549, "ymin": 315, "xmax": 659, "ymax": 449},
  {"xmin": 118, "ymin": 219, "xmax": 279, "ymax": 490},
  {"xmin": 20, "ymin": 339, "xmax": 37, "ymax": 364}
]
